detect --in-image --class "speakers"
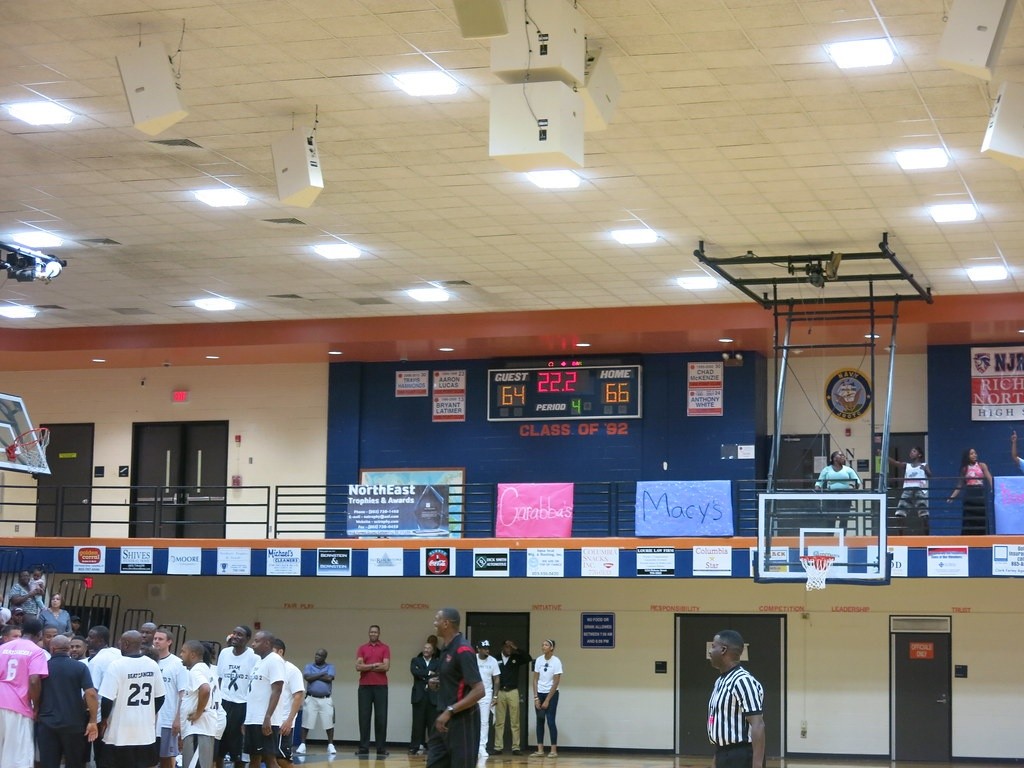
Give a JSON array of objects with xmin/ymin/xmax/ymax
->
[
  {"xmin": 271, "ymin": 126, "xmax": 323, "ymax": 208},
  {"xmin": 488, "ymin": 80, "xmax": 584, "ymax": 173},
  {"xmin": 115, "ymin": 40, "xmax": 189, "ymax": 137},
  {"xmin": 939, "ymin": 0, "xmax": 1017, "ymax": 81},
  {"xmin": 491, "ymin": 0, "xmax": 587, "ymax": 89},
  {"xmin": 980, "ymin": 82, "xmax": 1024, "ymax": 170}
]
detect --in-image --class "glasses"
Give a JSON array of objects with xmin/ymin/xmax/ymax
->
[{"xmin": 544, "ymin": 662, "xmax": 549, "ymax": 672}]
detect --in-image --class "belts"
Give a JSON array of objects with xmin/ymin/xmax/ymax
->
[{"xmin": 306, "ymin": 691, "xmax": 330, "ymax": 698}]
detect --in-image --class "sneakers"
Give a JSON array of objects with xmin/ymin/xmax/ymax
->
[
  {"xmin": 295, "ymin": 743, "xmax": 307, "ymax": 755},
  {"xmin": 328, "ymin": 743, "xmax": 337, "ymax": 754}
]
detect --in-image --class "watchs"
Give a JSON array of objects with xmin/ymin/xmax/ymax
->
[{"xmin": 447, "ymin": 705, "xmax": 456, "ymax": 716}]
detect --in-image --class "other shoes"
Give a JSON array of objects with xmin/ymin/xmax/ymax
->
[
  {"xmin": 355, "ymin": 751, "xmax": 369, "ymax": 754},
  {"xmin": 547, "ymin": 752, "xmax": 558, "ymax": 758},
  {"xmin": 408, "ymin": 748, "xmax": 418, "ymax": 754},
  {"xmin": 512, "ymin": 749, "xmax": 524, "ymax": 756},
  {"xmin": 487, "ymin": 748, "xmax": 504, "ymax": 755},
  {"xmin": 377, "ymin": 751, "xmax": 389, "ymax": 755},
  {"xmin": 530, "ymin": 751, "xmax": 545, "ymax": 757}
]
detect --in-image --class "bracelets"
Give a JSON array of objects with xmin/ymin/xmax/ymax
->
[
  {"xmin": 493, "ymin": 695, "xmax": 498, "ymax": 698},
  {"xmin": 534, "ymin": 696, "xmax": 539, "ymax": 700}
]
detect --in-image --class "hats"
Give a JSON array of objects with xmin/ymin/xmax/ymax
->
[
  {"xmin": 479, "ymin": 639, "xmax": 491, "ymax": 647},
  {"xmin": 226, "ymin": 634, "xmax": 233, "ymax": 642},
  {"xmin": 12, "ymin": 608, "xmax": 25, "ymax": 616},
  {"xmin": 71, "ymin": 616, "xmax": 81, "ymax": 621}
]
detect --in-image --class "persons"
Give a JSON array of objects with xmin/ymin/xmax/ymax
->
[
  {"xmin": 433, "ymin": 606, "xmax": 485, "ymax": 768},
  {"xmin": 408, "ymin": 636, "xmax": 563, "ymax": 757},
  {"xmin": 295, "ymin": 647, "xmax": 337, "ymax": 754},
  {"xmin": 707, "ymin": 629, "xmax": 766, "ymax": 768},
  {"xmin": 1008, "ymin": 428, "xmax": 1024, "ymax": 478},
  {"xmin": 814, "ymin": 450, "xmax": 862, "ymax": 536},
  {"xmin": 355, "ymin": 625, "xmax": 390, "ymax": 755},
  {"xmin": 877, "ymin": 445, "xmax": 932, "ymax": 536},
  {"xmin": 0, "ymin": 566, "xmax": 306, "ymax": 768},
  {"xmin": 946, "ymin": 446, "xmax": 993, "ymax": 535}
]
